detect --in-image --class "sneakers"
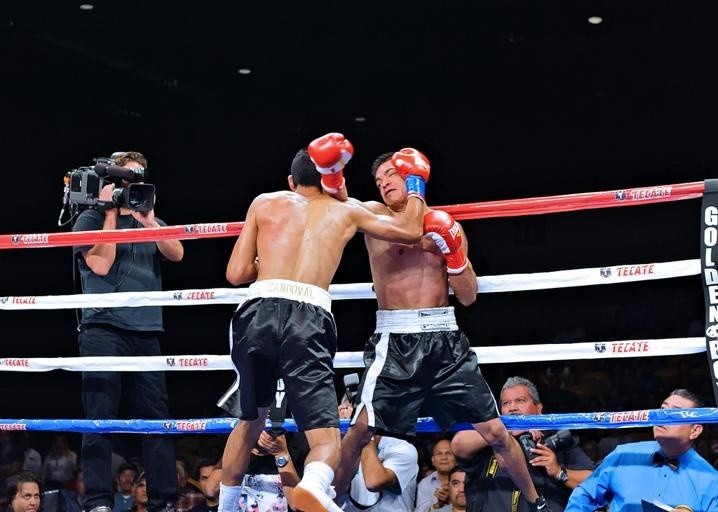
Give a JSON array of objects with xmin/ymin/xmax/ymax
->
[{"xmin": 290, "ymin": 483, "xmax": 344, "ymax": 511}]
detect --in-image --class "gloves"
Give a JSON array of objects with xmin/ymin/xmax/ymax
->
[
  {"xmin": 422, "ymin": 212, "xmax": 469, "ymax": 274},
  {"xmin": 308, "ymin": 133, "xmax": 355, "ymax": 193},
  {"xmin": 392, "ymin": 148, "xmax": 431, "ymax": 202}
]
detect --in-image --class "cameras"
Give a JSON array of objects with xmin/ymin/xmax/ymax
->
[
  {"xmin": 343, "ymin": 373, "xmax": 361, "ymax": 406},
  {"xmin": 519, "ymin": 429, "xmax": 579, "ymax": 466}
]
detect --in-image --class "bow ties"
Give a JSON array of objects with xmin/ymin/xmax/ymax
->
[{"xmin": 652, "ymin": 451, "xmax": 679, "ymax": 472}]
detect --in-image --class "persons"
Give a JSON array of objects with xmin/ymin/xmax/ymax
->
[
  {"xmin": 70, "ymin": 149, "xmax": 186, "ymax": 512},
  {"xmin": 306, "ymin": 133, "xmax": 558, "ymax": 511},
  {"xmin": 1, "ymin": 375, "xmax": 717, "ymax": 512},
  {"xmin": 216, "ymin": 147, "xmax": 432, "ymax": 512}
]
